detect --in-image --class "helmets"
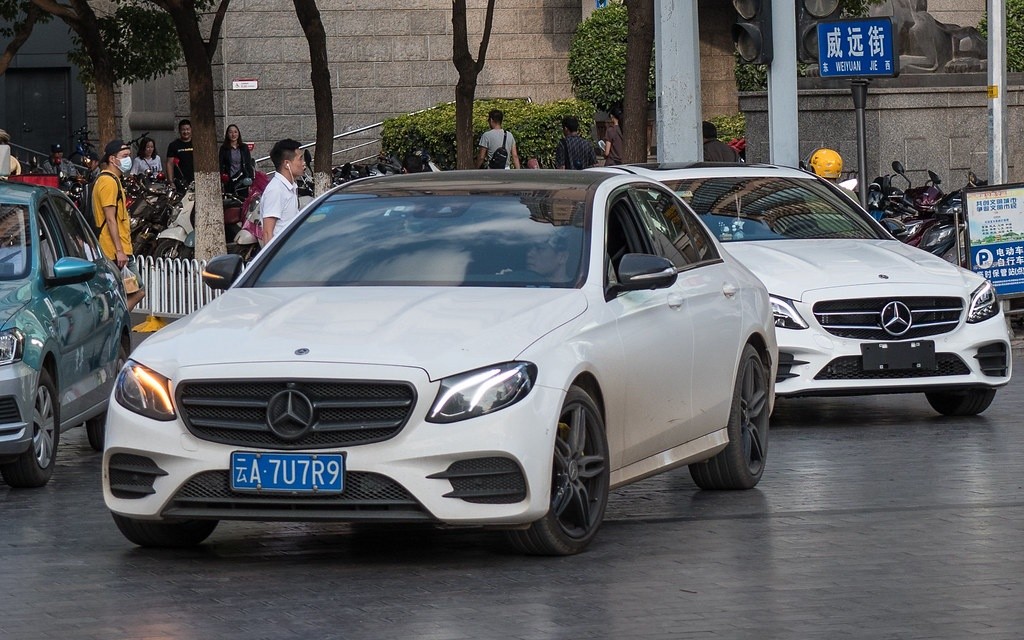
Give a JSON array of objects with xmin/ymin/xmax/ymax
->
[{"xmin": 810, "ymin": 148, "xmax": 843, "ymax": 178}]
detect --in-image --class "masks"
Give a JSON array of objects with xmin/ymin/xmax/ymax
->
[{"xmin": 113, "ymin": 157, "xmax": 132, "ymax": 174}]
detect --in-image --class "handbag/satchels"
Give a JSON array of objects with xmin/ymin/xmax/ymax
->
[
  {"xmin": 120, "ymin": 265, "xmax": 141, "ymax": 294},
  {"xmin": 489, "ymin": 146, "xmax": 508, "ymax": 170}
]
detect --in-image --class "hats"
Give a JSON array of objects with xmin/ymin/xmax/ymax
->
[
  {"xmin": 51, "ymin": 143, "xmax": 64, "ymax": 153},
  {"xmin": 100, "ymin": 139, "xmax": 130, "ymax": 163},
  {"xmin": 85, "ymin": 152, "xmax": 99, "ymax": 160}
]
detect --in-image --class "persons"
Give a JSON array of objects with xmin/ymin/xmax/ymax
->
[
  {"xmin": 601, "ymin": 108, "xmax": 624, "ymax": 167},
  {"xmin": 92, "ymin": 140, "xmax": 146, "ymax": 313},
  {"xmin": 495, "ymin": 230, "xmax": 571, "ymax": 288},
  {"xmin": 87, "ymin": 153, "xmax": 100, "ymax": 181},
  {"xmin": 402, "ymin": 154, "xmax": 424, "ymax": 174},
  {"xmin": 130, "ymin": 137, "xmax": 163, "ymax": 178},
  {"xmin": 476, "ymin": 109, "xmax": 520, "ymax": 170},
  {"xmin": 809, "ymin": 148, "xmax": 860, "ymax": 205},
  {"xmin": 43, "ymin": 144, "xmax": 76, "ymax": 177},
  {"xmin": 702, "ymin": 121, "xmax": 740, "ymax": 163},
  {"xmin": 165, "ymin": 119, "xmax": 195, "ymax": 192},
  {"xmin": 260, "ymin": 138, "xmax": 305, "ymax": 246},
  {"xmin": 219, "ymin": 124, "xmax": 251, "ymax": 196},
  {"xmin": 556, "ymin": 115, "xmax": 598, "ymax": 169}
]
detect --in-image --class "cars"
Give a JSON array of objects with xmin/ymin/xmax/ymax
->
[
  {"xmin": 585, "ymin": 162, "xmax": 1013, "ymax": 417},
  {"xmin": 100, "ymin": 169, "xmax": 782, "ymax": 558},
  {"xmin": 1, "ymin": 179, "xmax": 132, "ymax": 486}
]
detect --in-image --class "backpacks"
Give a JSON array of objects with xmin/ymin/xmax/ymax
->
[{"xmin": 79, "ymin": 172, "xmax": 126, "ymax": 241}]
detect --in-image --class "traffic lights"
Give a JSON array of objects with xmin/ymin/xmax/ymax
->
[
  {"xmin": 727, "ymin": 0, "xmax": 772, "ymax": 65},
  {"xmin": 795, "ymin": 0, "xmax": 839, "ymax": 66}
]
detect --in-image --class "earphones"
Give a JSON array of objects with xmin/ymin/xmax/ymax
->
[{"xmin": 286, "ymin": 164, "xmax": 290, "ymax": 168}]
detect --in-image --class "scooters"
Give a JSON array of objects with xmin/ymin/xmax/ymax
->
[
  {"xmin": 33, "ymin": 126, "xmax": 449, "ymax": 263},
  {"xmin": 866, "ymin": 162, "xmax": 990, "ymax": 268}
]
